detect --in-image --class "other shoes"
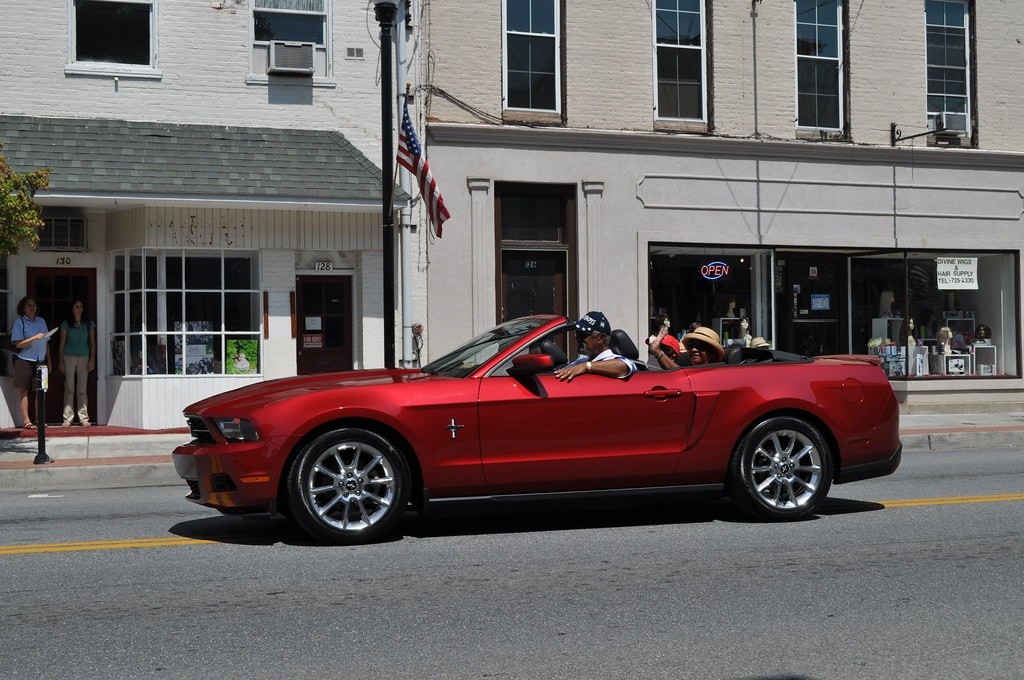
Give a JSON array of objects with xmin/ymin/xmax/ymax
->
[
  {"xmin": 79, "ymin": 421, "xmax": 91, "ymax": 427},
  {"xmin": 62, "ymin": 421, "xmax": 73, "ymax": 427}
]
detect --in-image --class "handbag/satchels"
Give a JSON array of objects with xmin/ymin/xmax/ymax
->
[{"xmin": 0, "ymin": 317, "xmax": 26, "ymax": 354}]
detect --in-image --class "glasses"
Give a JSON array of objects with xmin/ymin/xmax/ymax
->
[
  {"xmin": 575, "ymin": 330, "xmax": 593, "ymax": 337},
  {"xmin": 686, "ymin": 341, "xmax": 711, "ymax": 352}
]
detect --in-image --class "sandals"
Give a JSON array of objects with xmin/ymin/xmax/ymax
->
[
  {"xmin": 23, "ymin": 422, "xmax": 37, "ymax": 429},
  {"xmin": 34, "ymin": 421, "xmax": 47, "ymax": 428}
]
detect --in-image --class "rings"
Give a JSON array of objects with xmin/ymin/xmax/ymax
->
[{"xmin": 567, "ymin": 371, "xmax": 571, "ymax": 374}]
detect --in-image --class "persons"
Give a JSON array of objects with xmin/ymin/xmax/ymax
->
[
  {"xmin": 11, "ymin": 296, "xmax": 51, "ymax": 429},
  {"xmin": 738, "ymin": 316, "xmax": 751, "ymax": 339},
  {"xmin": 109, "ymin": 341, "xmax": 125, "ymax": 375},
  {"xmin": 175, "ymin": 321, "xmax": 214, "ymax": 375},
  {"xmin": 653, "ymin": 314, "xmax": 670, "ymax": 334},
  {"xmin": 56, "ymin": 300, "xmax": 96, "ymax": 428},
  {"xmin": 942, "ymin": 290, "xmax": 960, "ymax": 311},
  {"xmin": 879, "ymin": 290, "xmax": 894, "ymax": 318},
  {"xmin": 937, "ymin": 326, "xmax": 953, "ymax": 354},
  {"xmin": 722, "ymin": 294, "xmax": 738, "ymax": 317},
  {"xmin": 645, "ymin": 333, "xmax": 680, "ymax": 366},
  {"xmin": 553, "ymin": 311, "xmax": 637, "ymax": 380},
  {"xmin": 651, "ymin": 324, "xmax": 724, "ymax": 369},
  {"xmin": 233, "ymin": 352, "xmax": 252, "ymax": 371},
  {"xmin": 974, "ymin": 324, "xmax": 992, "ymax": 338},
  {"xmin": 132, "ymin": 350, "xmax": 157, "ymax": 375},
  {"xmin": 904, "ymin": 317, "xmax": 918, "ymax": 346}
]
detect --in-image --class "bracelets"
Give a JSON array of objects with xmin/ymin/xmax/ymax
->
[
  {"xmin": 653, "ymin": 350, "xmax": 664, "ymax": 359},
  {"xmin": 585, "ymin": 361, "xmax": 595, "ymax": 373}
]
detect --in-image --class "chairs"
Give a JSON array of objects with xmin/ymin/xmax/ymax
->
[
  {"xmin": 608, "ymin": 329, "xmax": 650, "ymax": 371},
  {"xmin": 527, "ymin": 337, "xmax": 571, "ymax": 375},
  {"xmin": 673, "ymin": 345, "xmax": 742, "ymax": 369}
]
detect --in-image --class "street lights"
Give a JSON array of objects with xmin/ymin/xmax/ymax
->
[{"xmin": 371, "ymin": 0, "xmax": 398, "ymax": 369}]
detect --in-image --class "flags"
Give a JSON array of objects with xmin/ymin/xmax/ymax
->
[{"xmin": 396, "ymin": 101, "xmax": 451, "ymax": 239}]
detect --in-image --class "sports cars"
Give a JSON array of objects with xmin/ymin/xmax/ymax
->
[{"xmin": 171, "ymin": 313, "xmax": 905, "ymax": 548}]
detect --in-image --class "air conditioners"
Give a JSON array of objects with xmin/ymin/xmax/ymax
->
[
  {"xmin": 265, "ymin": 39, "xmax": 317, "ymax": 76},
  {"xmin": 933, "ymin": 111, "xmax": 970, "ymax": 139}
]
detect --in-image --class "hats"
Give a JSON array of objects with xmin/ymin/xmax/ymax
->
[
  {"xmin": 572, "ymin": 311, "xmax": 611, "ymax": 336},
  {"xmin": 645, "ymin": 334, "xmax": 681, "ymax": 359},
  {"xmin": 681, "ymin": 326, "xmax": 725, "ymax": 362},
  {"xmin": 749, "ymin": 337, "xmax": 771, "ymax": 349}
]
